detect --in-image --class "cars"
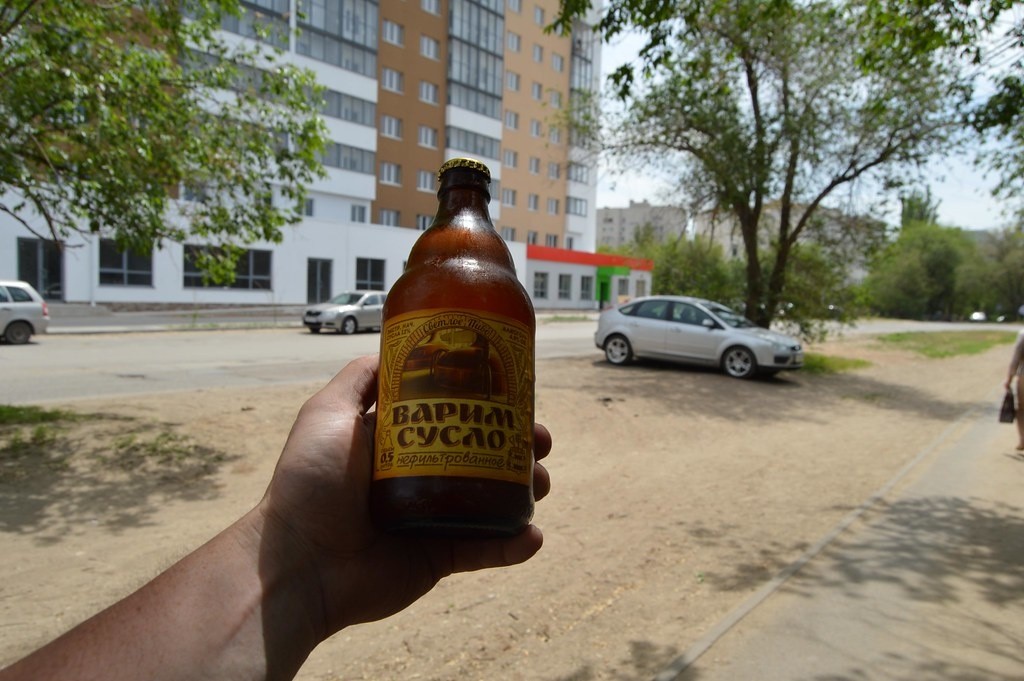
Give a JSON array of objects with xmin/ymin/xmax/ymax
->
[
  {"xmin": 595, "ymin": 294, "xmax": 805, "ymax": 380},
  {"xmin": 302, "ymin": 290, "xmax": 388, "ymax": 336}
]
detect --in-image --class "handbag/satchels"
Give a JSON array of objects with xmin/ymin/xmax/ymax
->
[{"xmin": 1000, "ymin": 386, "xmax": 1016, "ymax": 422}]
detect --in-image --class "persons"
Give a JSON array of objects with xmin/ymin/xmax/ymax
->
[
  {"xmin": 1005, "ymin": 304, "xmax": 1024, "ymax": 451},
  {"xmin": 0, "ymin": 357, "xmax": 551, "ymax": 681}
]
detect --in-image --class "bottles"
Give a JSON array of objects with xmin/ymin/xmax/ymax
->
[{"xmin": 371, "ymin": 158, "xmax": 535, "ymax": 536}]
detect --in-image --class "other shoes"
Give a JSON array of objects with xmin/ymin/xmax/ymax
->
[{"xmin": 1017, "ymin": 443, "xmax": 1024, "ymax": 450}]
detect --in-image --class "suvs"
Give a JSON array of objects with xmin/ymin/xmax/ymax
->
[{"xmin": 1, "ymin": 279, "xmax": 51, "ymax": 345}]
